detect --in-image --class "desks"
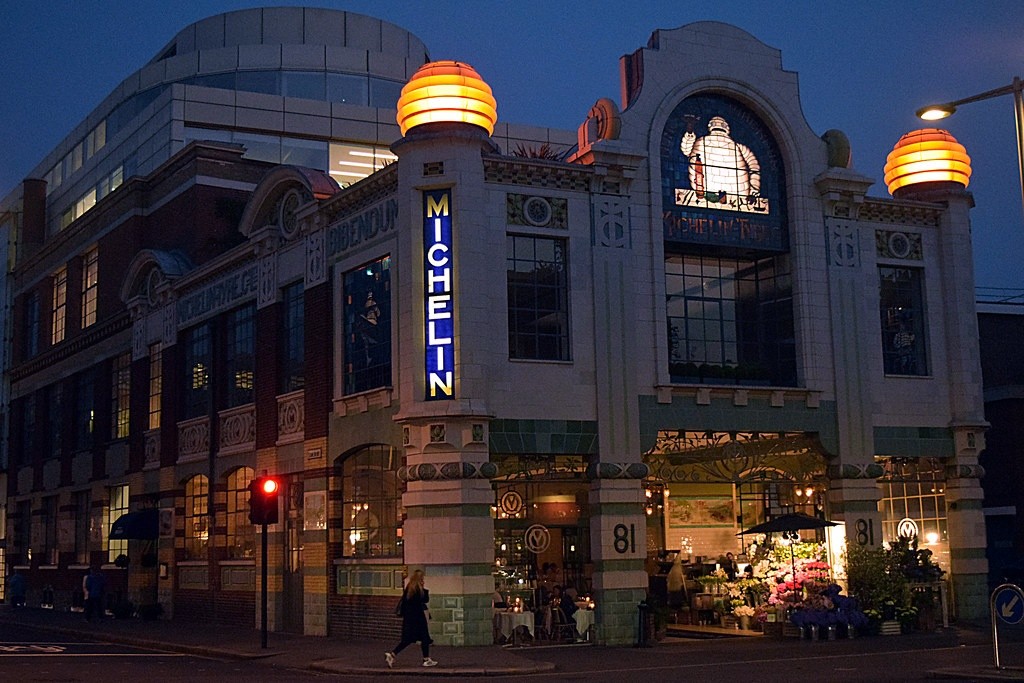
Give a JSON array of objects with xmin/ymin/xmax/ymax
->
[
  {"xmin": 572, "ymin": 609, "xmax": 595, "ymax": 642},
  {"xmin": 495, "ymin": 610, "xmax": 534, "ymax": 644}
]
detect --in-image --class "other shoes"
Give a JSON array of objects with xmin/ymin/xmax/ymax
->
[{"xmin": 577, "ymin": 639, "xmax": 586, "ymax": 642}]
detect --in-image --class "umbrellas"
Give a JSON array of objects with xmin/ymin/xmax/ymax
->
[{"xmin": 735, "ymin": 512, "xmax": 844, "ymax": 602}]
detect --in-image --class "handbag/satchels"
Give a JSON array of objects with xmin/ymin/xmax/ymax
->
[{"xmin": 396, "ymin": 594, "xmax": 403, "ymax": 618}]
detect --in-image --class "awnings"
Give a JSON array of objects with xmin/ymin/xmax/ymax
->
[{"xmin": 108, "ymin": 508, "xmax": 160, "ymax": 540}]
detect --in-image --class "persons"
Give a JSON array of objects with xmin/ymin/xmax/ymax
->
[
  {"xmin": 82, "ymin": 564, "xmax": 107, "ymax": 624},
  {"xmin": 549, "ymin": 585, "xmax": 575, "ymax": 644},
  {"xmin": 385, "ymin": 570, "xmax": 438, "ymax": 670},
  {"xmin": 726, "ymin": 552, "xmax": 739, "ymax": 582},
  {"xmin": 494, "ymin": 582, "xmax": 524, "ymax": 644}
]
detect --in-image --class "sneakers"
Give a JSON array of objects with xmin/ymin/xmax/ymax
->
[
  {"xmin": 384, "ymin": 651, "xmax": 396, "ymax": 669},
  {"xmin": 422, "ymin": 657, "xmax": 438, "ymax": 666}
]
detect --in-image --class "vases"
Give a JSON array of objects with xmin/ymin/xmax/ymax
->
[
  {"xmin": 711, "ymin": 584, "xmax": 718, "ymax": 593},
  {"xmin": 827, "ymin": 623, "xmax": 838, "ymax": 641},
  {"xmin": 811, "ymin": 621, "xmax": 820, "ymax": 642},
  {"xmin": 702, "ymin": 585, "xmax": 710, "ymax": 593},
  {"xmin": 797, "ymin": 622, "xmax": 807, "ymax": 640},
  {"xmin": 876, "ymin": 619, "xmax": 900, "ymax": 637},
  {"xmin": 846, "ymin": 622, "xmax": 856, "ymax": 640},
  {"xmin": 739, "ymin": 615, "xmax": 752, "ymax": 630}
]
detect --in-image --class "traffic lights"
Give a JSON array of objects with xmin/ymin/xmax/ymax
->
[{"xmin": 248, "ymin": 476, "xmax": 279, "ymax": 525}]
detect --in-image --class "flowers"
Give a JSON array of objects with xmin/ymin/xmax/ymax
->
[{"xmin": 701, "ymin": 532, "xmax": 942, "ymax": 623}]
